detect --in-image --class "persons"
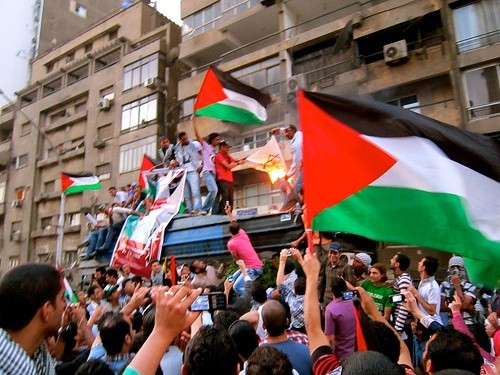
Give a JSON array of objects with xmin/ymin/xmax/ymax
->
[
  {"xmin": 147, "ymin": 115, "xmax": 250, "ymax": 216},
  {"xmin": 272, "ymin": 125, "xmax": 303, "ymax": 201},
  {"xmin": 0, "ymin": 259, "xmax": 241, "ymax": 375},
  {"xmin": 80, "ymin": 182, "xmax": 152, "ymax": 260},
  {"xmin": 224, "ymin": 203, "xmax": 500, "ymax": 375}
]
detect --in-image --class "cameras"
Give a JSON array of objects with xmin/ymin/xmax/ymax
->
[
  {"xmin": 389, "ymin": 294, "xmax": 405, "ymax": 303},
  {"xmin": 341, "ymin": 290, "xmax": 357, "ymax": 300},
  {"xmin": 288, "ymin": 249, "xmax": 292, "ymax": 256}
]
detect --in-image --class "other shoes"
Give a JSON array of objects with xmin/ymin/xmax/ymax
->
[
  {"xmin": 190, "ymin": 209, "xmax": 198, "ymax": 214},
  {"xmin": 199, "ymin": 211, "xmax": 208, "ymax": 215}
]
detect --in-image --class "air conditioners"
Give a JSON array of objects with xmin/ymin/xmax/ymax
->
[
  {"xmin": 97, "ymin": 98, "xmax": 111, "ymax": 111},
  {"xmin": 12, "ymin": 199, "xmax": 22, "ymax": 208},
  {"xmin": 40, "ymin": 192, "xmax": 49, "ymax": 199},
  {"xmin": 287, "ymin": 73, "xmax": 307, "ymax": 94},
  {"xmin": 145, "ymin": 77, "xmax": 156, "ymax": 88},
  {"xmin": 383, "ymin": 40, "xmax": 407, "ymax": 64},
  {"xmin": 93, "ymin": 141, "xmax": 105, "ymax": 147},
  {"xmin": 59, "ymin": 149, "xmax": 66, "ymax": 154},
  {"xmin": 12, "ymin": 233, "xmax": 21, "ymax": 241}
]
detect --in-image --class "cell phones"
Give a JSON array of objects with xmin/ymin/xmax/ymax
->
[{"xmin": 190, "ymin": 292, "xmax": 226, "ymax": 312}]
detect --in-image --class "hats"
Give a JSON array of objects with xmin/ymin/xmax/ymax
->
[
  {"xmin": 356, "ymin": 253, "xmax": 372, "ymax": 268},
  {"xmin": 449, "ymin": 256, "xmax": 464, "ymax": 268},
  {"xmin": 327, "ymin": 242, "xmax": 342, "ymax": 252},
  {"xmin": 104, "ymin": 284, "xmax": 119, "ymax": 296},
  {"xmin": 220, "ymin": 140, "xmax": 232, "ymax": 148},
  {"xmin": 228, "ymin": 320, "xmax": 260, "ymax": 359}
]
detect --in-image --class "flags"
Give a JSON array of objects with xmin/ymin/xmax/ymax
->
[
  {"xmin": 61, "ymin": 172, "xmax": 101, "ymax": 195},
  {"xmin": 58, "ymin": 262, "xmax": 78, "ymax": 303},
  {"xmin": 244, "ymin": 135, "xmax": 288, "ymax": 184},
  {"xmin": 194, "ymin": 65, "xmax": 271, "ymax": 124},
  {"xmin": 298, "ymin": 87, "xmax": 500, "ymax": 291},
  {"xmin": 139, "ymin": 155, "xmax": 155, "ymax": 189}
]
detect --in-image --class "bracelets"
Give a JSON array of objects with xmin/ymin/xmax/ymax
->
[{"xmin": 237, "ymin": 162, "xmax": 239, "ymax": 164}]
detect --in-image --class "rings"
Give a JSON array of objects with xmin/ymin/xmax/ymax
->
[{"xmin": 164, "ymin": 291, "xmax": 174, "ymax": 296}]
